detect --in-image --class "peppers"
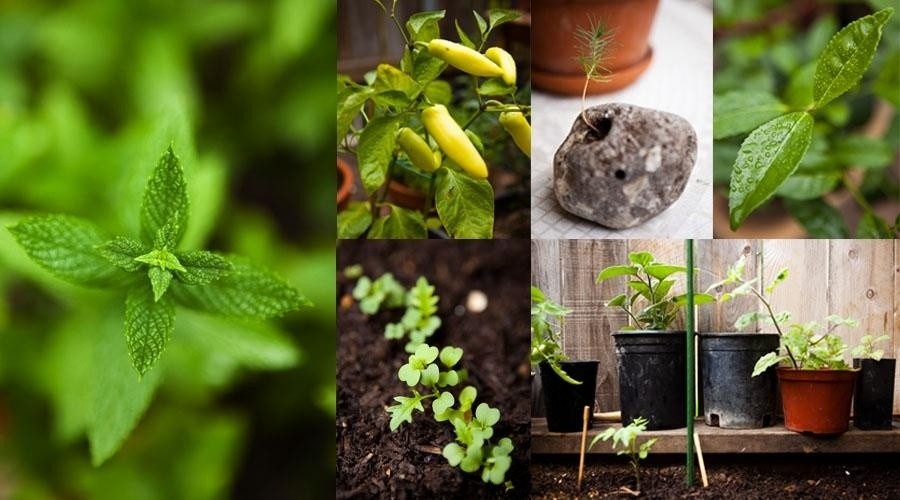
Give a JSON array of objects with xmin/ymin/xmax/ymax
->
[{"xmin": 396, "ymin": 37, "xmax": 531, "ymax": 180}]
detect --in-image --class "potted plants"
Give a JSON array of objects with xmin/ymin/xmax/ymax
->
[{"xmin": 530, "ymin": 247, "xmax": 898, "ymax": 439}]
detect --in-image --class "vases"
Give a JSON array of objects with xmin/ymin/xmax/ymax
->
[{"xmin": 533, "ymin": 0, "xmax": 662, "ymax": 99}]
